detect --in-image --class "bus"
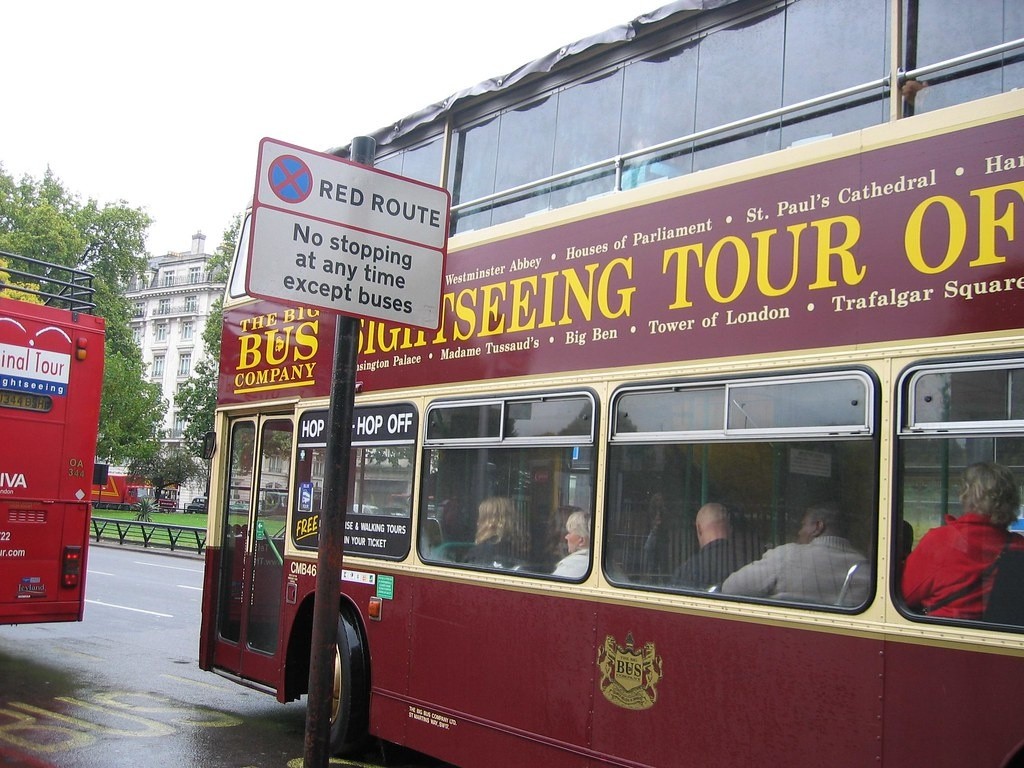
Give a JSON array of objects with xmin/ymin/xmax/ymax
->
[
  {"xmin": 1, "ymin": 253, "xmax": 106, "ymax": 628},
  {"xmin": 195, "ymin": 0, "xmax": 1024, "ymax": 768}
]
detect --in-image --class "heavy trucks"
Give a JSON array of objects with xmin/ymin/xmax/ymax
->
[{"xmin": 91, "ymin": 462, "xmax": 178, "ymax": 516}]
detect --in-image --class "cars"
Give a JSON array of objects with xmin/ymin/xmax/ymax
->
[
  {"xmin": 352, "ymin": 503, "xmax": 382, "ymax": 515},
  {"xmin": 229, "ymin": 500, "xmax": 251, "ymax": 512}
]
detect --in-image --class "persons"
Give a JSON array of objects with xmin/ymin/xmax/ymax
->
[
  {"xmin": 620, "ymin": 139, "xmax": 689, "ymax": 191},
  {"xmin": 385, "ymin": 461, "xmax": 1024, "ymax": 623}
]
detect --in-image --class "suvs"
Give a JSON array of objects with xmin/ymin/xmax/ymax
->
[{"xmin": 187, "ymin": 496, "xmax": 209, "ymax": 514}]
